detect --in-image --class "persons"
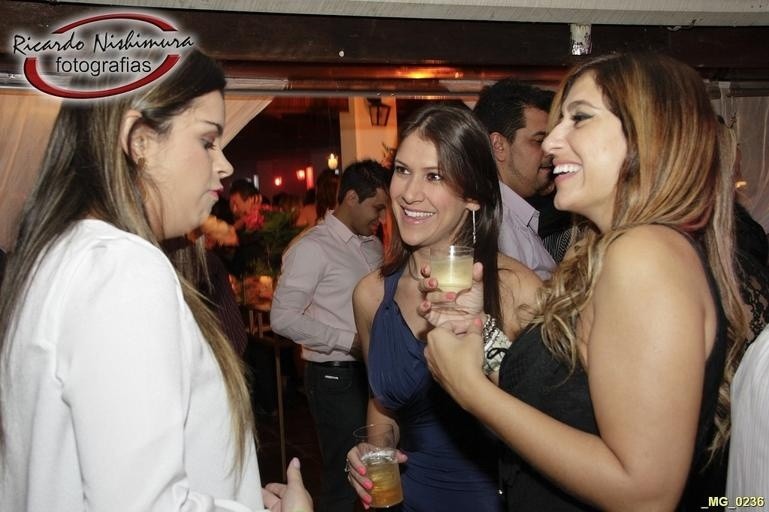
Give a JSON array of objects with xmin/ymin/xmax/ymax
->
[
  {"xmin": 418, "ymin": 50, "xmax": 749, "ymax": 512},
  {"xmin": 0, "ymin": 33, "xmax": 315, "ymax": 512},
  {"xmin": 715, "ymin": 115, "xmax": 768, "ymax": 510},
  {"xmin": 472, "ymin": 77, "xmax": 555, "ymax": 280},
  {"xmin": 342, "ymin": 101, "xmax": 552, "ymax": 512},
  {"xmin": 228, "ymin": 168, "xmax": 341, "ymax": 231},
  {"xmin": 268, "ymin": 161, "xmax": 393, "ymax": 512}
]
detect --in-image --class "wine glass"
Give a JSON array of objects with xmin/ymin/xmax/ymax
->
[{"xmin": 430, "ymin": 245, "xmax": 474, "ymax": 308}]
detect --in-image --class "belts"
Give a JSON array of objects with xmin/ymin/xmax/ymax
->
[{"xmin": 322, "ymin": 361, "xmax": 359, "ymax": 367}]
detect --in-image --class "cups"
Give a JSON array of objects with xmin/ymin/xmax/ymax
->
[{"xmin": 353, "ymin": 423, "xmax": 403, "ymax": 508}]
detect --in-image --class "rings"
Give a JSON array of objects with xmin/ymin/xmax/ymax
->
[{"xmin": 342, "ymin": 461, "xmax": 352, "ymax": 473}]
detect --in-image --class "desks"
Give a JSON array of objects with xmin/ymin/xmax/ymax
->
[{"xmin": 235, "ymin": 302, "xmax": 303, "ymax": 484}]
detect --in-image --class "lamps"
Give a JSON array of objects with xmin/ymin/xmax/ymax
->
[{"xmin": 367, "ymin": 97, "xmax": 391, "ymax": 126}]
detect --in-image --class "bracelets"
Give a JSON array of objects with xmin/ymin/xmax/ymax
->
[{"xmin": 480, "ymin": 315, "xmax": 513, "ymax": 375}]
